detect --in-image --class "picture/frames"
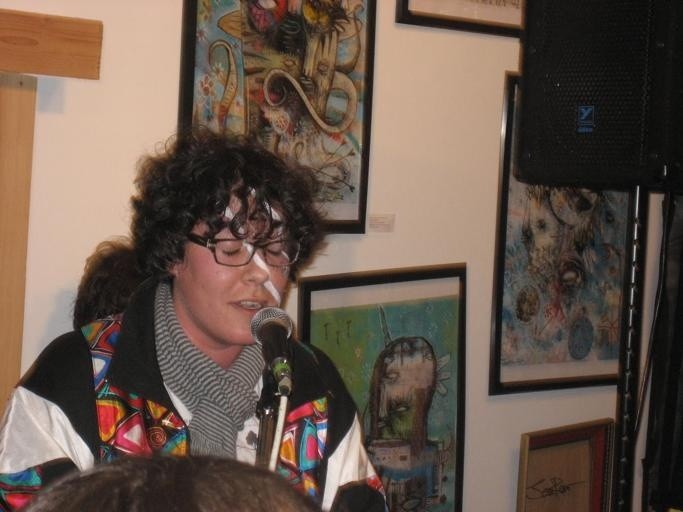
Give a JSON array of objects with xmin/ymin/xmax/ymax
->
[
  {"xmin": 394, "ymin": 2, "xmax": 524, "ymax": 39},
  {"xmin": 489, "ymin": 72, "xmax": 629, "ymax": 396},
  {"xmin": 178, "ymin": 1, "xmax": 377, "ymax": 234},
  {"xmin": 295, "ymin": 262, "xmax": 466, "ymax": 512},
  {"xmin": 517, "ymin": 418, "xmax": 615, "ymax": 512}
]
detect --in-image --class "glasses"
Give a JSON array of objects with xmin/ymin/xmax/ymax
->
[{"xmin": 188, "ymin": 232, "xmax": 300, "ymax": 266}]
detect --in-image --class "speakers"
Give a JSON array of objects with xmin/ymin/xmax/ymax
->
[{"xmin": 513, "ymin": 0, "xmax": 682, "ymax": 195}]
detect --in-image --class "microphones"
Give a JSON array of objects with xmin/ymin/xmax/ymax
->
[{"xmin": 250, "ymin": 308, "xmax": 292, "ymax": 396}]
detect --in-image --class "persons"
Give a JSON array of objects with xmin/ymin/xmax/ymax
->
[
  {"xmin": 13, "ymin": 451, "xmax": 324, "ymax": 512},
  {"xmin": 72, "ymin": 234, "xmax": 141, "ymax": 329},
  {"xmin": 0, "ymin": 124, "xmax": 388, "ymax": 512}
]
